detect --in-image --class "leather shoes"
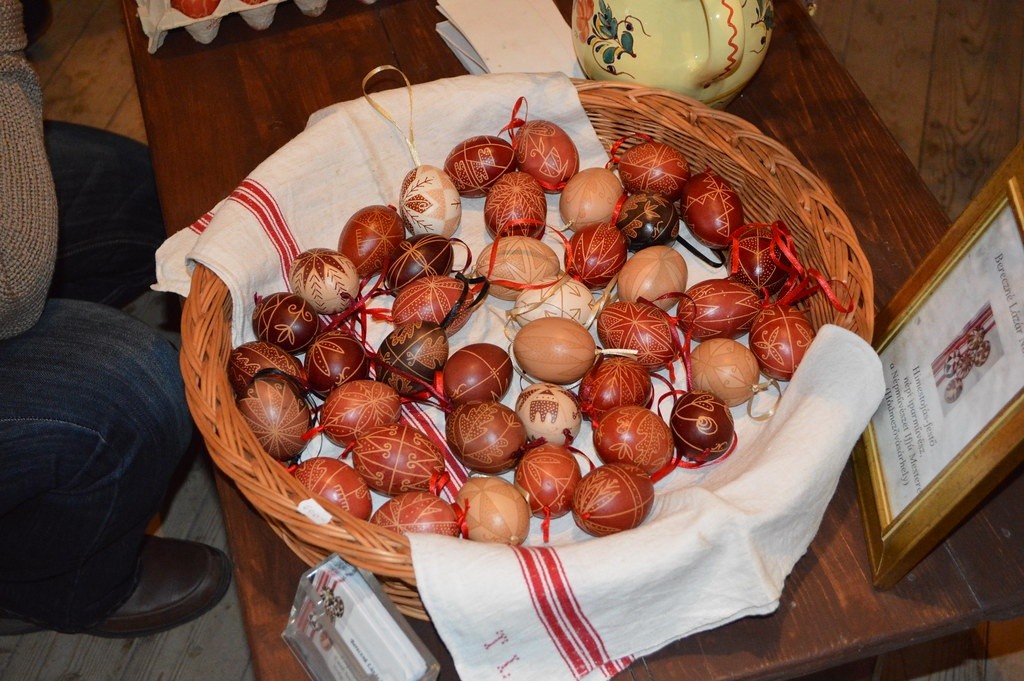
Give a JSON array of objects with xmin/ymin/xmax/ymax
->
[{"xmin": 0, "ymin": 534, "xmax": 231, "ymax": 639}]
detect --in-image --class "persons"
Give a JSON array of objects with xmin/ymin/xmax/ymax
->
[{"xmin": 0, "ymin": 0, "xmax": 233, "ymax": 638}]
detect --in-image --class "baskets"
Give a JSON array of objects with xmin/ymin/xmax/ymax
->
[{"xmin": 179, "ymin": 69, "xmax": 874, "ymax": 625}]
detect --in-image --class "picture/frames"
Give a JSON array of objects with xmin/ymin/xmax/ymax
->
[{"xmin": 845, "ymin": 132, "xmax": 1024, "ymax": 585}]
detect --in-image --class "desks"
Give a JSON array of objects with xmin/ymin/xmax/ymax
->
[{"xmin": 118, "ymin": 0, "xmax": 1024, "ymax": 681}]
